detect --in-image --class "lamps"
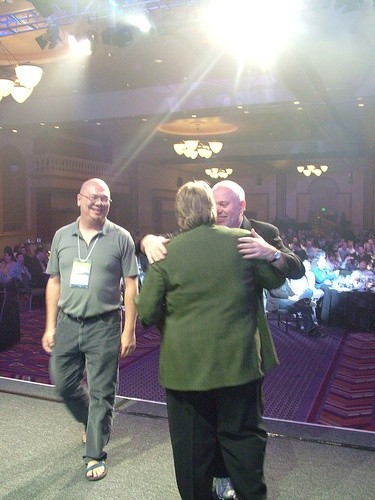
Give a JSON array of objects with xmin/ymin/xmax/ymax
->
[
  {"xmin": 173, "ymin": 121, "xmax": 223, "ymax": 159},
  {"xmin": 205, "ymin": 161, "xmax": 234, "ymax": 179},
  {"xmin": 0, "ymin": 41, "xmax": 43, "ymax": 104},
  {"xmin": 297, "ymin": 142, "xmax": 328, "ymax": 177}
]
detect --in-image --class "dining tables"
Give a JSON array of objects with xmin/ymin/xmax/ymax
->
[{"xmin": 320, "ymin": 283, "xmax": 375, "ymax": 328}]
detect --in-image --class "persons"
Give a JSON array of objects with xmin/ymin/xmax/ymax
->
[
  {"xmin": 133, "ymin": 180, "xmax": 306, "ymax": 500},
  {"xmin": 0, "ymin": 244, "xmax": 50, "ymax": 308},
  {"xmin": 40, "ymin": 181, "xmax": 140, "ymax": 481},
  {"xmin": 133, "ymin": 180, "xmax": 285, "ymax": 499},
  {"xmin": 119, "ymin": 256, "xmax": 145, "ymax": 307},
  {"xmin": 265, "ymin": 228, "xmax": 375, "ymax": 338}
]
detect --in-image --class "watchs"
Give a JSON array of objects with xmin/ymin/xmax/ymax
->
[{"xmin": 269, "ymin": 250, "xmax": 281, "ymax": 263}]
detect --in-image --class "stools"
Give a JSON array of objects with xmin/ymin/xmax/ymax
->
[{"xmin": 278, "ymin": 308, "xmax": 299, "ymax": 332}]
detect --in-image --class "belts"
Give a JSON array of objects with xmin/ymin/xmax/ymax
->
[{"xmin": 61, "ymin": 307, "xmax": 124, "ymax": 323}]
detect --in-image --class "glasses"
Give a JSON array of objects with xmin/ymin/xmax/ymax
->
[{"xmin": 80, "ymin": 193, "xmax": 112, "ymax": 203}]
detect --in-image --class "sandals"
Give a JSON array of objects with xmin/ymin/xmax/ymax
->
[
  {"xmin": 85, "ymin": 457, "xmax": 107, "ymax": 481},
  {"xmin": 82, "ymin": 431, "xmax": 86, "ymax": 444}
]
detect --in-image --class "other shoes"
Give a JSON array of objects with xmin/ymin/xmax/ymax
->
[
  {"xmin": 212, "ymin": 477, "xmax": 236, "ymax": 500},
  {"xmin": 320, "ymin": 320, "xmax": 330, "ymax": 327},
  {"xmin": 309, "ymin": 329, "xmax": 321, "ymax": 337}
]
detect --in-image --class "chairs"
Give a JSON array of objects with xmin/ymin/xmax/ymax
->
[{"xmin": 0, "ymin": 279, "xmax": 44, "ymax": 323}]
